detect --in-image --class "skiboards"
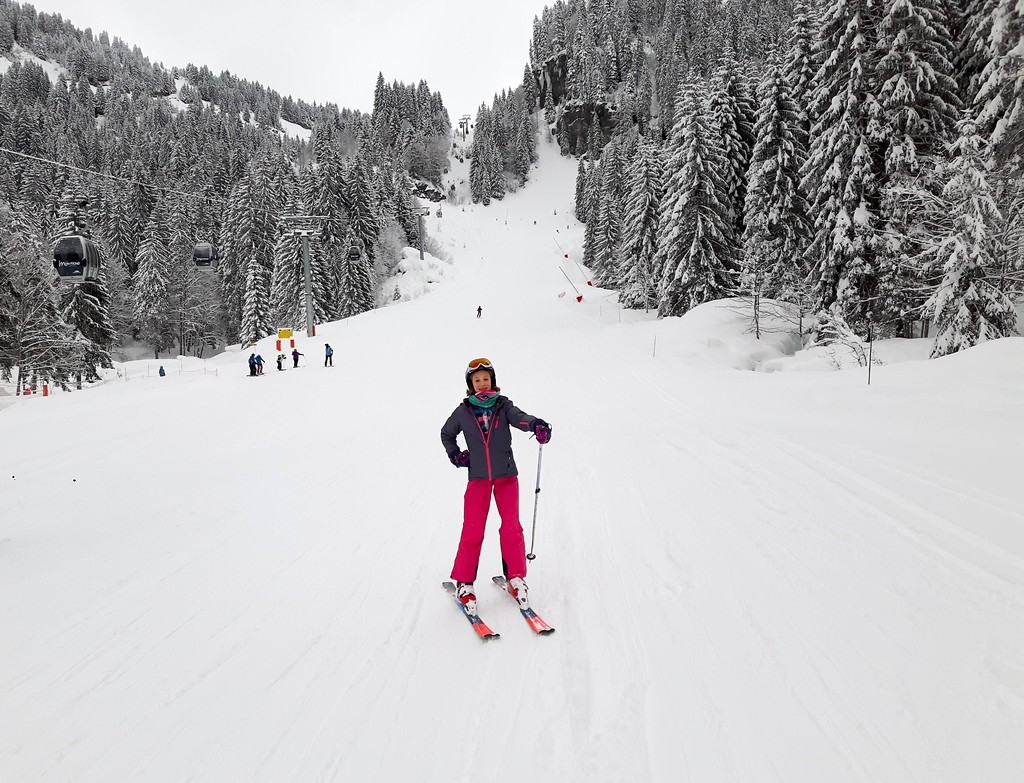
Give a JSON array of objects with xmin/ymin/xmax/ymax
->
[{"xmin": 441, "ymin": 575, "xmax": 555, "ymax": 640}]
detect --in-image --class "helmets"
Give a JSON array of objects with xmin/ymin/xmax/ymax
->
[{"xmin": 465, "ymin": 366, "xmax": 496, "ymax": 391}]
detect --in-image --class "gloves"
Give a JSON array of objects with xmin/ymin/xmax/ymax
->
[
  {"xmin": 451, "ymin": 450, "xmax": 470, "ymax": 468},
  {"xmin": 533, "ymin": 419, "xmax": 552, "ymax": 444}
]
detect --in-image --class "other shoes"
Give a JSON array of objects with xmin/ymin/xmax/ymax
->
[
  {"xmin": 457, "ymin": 582, "xmax": 476, "ymax": 604},
  {"xmin": 508, "ymin": 579, "xmax": 527, "ymax": 596}
]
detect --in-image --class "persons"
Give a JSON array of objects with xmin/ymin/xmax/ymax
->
[
  {"xmin": 440, "ymin": 358, "xmax": 551, "ymax": 616},
  {"xmin": 324, "ymin": 343, "xmax": 334, "ymax": 366},
  {"xmin": 248, "ymin": 353, "xmax": 257, "ymax": 375},
  {"xmin": 159, "ymin": 366, "xmax": 166, "ymax": 377},
  {"xmin": 291, "ymin": 349, "xmax": 304, "ymax": 367},
  {"xmin": 276, "ymin": 354, "xmax": 282, "ymax": 370},
  {"xmin": 255, "ymin": 355, "xmax": 265, "ymax": 374},
  {"xmin": 476, "ymin": 306, "xmax": 482, "ymax": 318}
]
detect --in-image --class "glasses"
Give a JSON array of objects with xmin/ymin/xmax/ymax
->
[{"xmin": 467, "ymin": 358, "xmax": 493, "ymax": 371}]
